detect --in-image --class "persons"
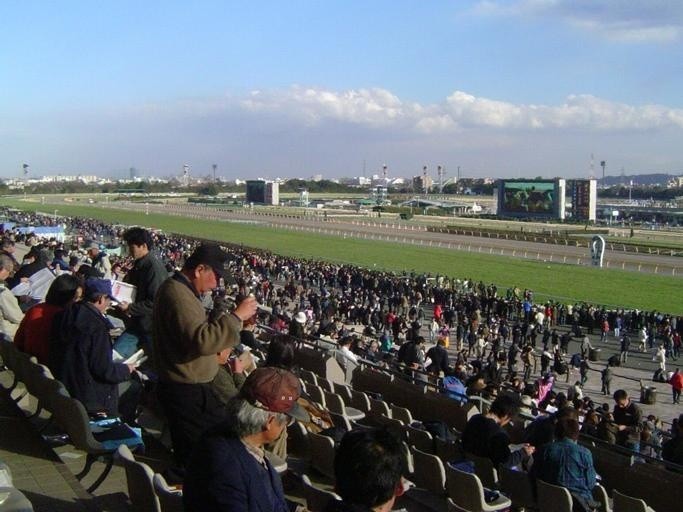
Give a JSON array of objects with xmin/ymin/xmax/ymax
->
[{"xmin": 1, "ymin": 206, "xmax": 682, "ymax": 510}]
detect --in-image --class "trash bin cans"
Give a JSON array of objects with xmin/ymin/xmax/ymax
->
[
  {"xmin": 641, "ymin": 385, "xmax": 657, "ymax": 404},
  {"xmin": 589, "ymin": 347, "xmax": 601, "ymax": 361}
]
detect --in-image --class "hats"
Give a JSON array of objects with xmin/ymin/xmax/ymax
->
[
  {"xmin": 241, "ymin": 367, "xmax": 310, "ymax": 423},
  {"xmin": 85, "ymin": 279, "xmax": 122, "ymax": 304},
  {"xmin": 295, "ymin": 312, "xmax": 306, "ymax": 323},
  {"xmin": 191, "ymin": 245, "xmax": 237, "ymax": 286}
]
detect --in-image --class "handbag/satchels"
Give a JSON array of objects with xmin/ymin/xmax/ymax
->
[{"xmin": 124, "ymin": 380, "xmax": 167, "ymax": 430}]
